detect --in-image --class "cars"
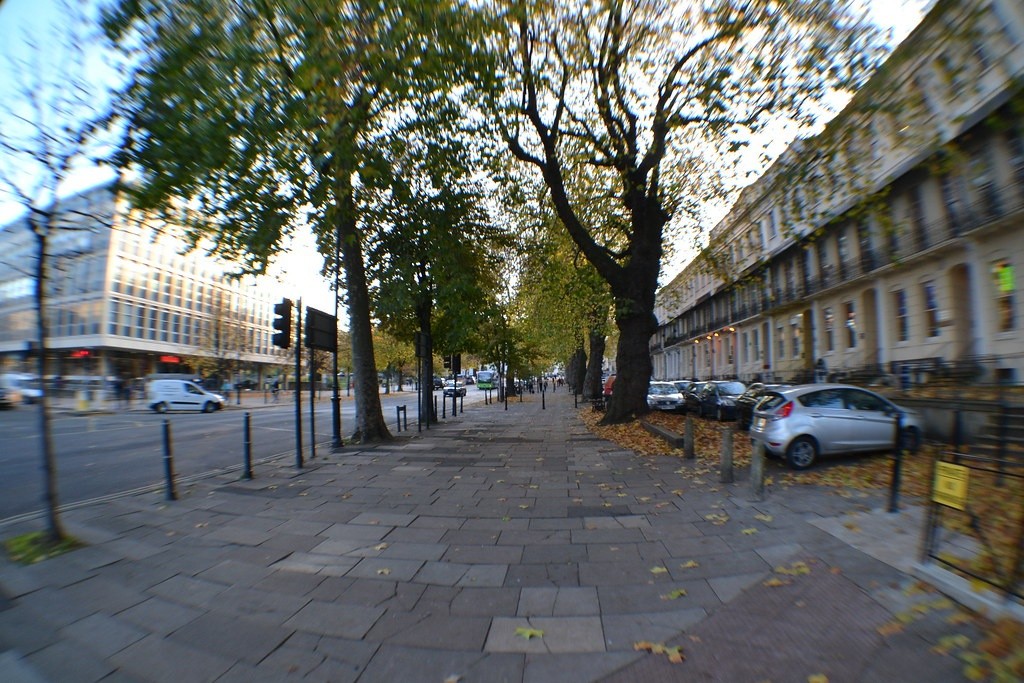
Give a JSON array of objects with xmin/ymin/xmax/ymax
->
[
  {"xmin": 0, "ymin": 387, "xmax": 19, "ymax": 410},
  {"xmin": 497, "ymin": 372, "xmax": 561, "ymax": 392},
  {"xmin": 601, "ymin": 369, "xmax": 618, "ymax": 404},
  {"xmin": 698, "ymin": 379, "xmax": 749, "ymax": 421},
  {"xmin": 736, "ymin": 380, "xmax": 795, "ymax": 432},
  {"xmin": 748, "ymin": 382, "xmax": 923, "ymax": 470},
  {"xmin": 672, "ymin": 379, "xmax": 692, "ymax": 395},
  {"xmin": 645, "ymin": 380, "xmax": 684, "ymax": 412},
  {"xmin": 680, "ymin": 382, "xmax": 709, "ymax": 414},
  {"xmin": 430, "ymin": 372, "xmax": 475, "ymax": 397}
]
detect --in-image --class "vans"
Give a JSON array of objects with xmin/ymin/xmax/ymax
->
[
  {"xmin": 0, "ymin": 373, "xmax": 45, "ymax": 402},
  {"xmin": 142, "ymin": 377, "xmax": 223, "ymax": 414}
]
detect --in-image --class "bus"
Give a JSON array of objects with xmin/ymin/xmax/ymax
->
[{"xmin": 477, "ymin": 370, "xmax": 499, "ymax": 389}]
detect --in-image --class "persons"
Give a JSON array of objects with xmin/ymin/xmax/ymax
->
[{"xmin": 521, "ymin": 373, "xmax": 564, "ymax": 395}]
